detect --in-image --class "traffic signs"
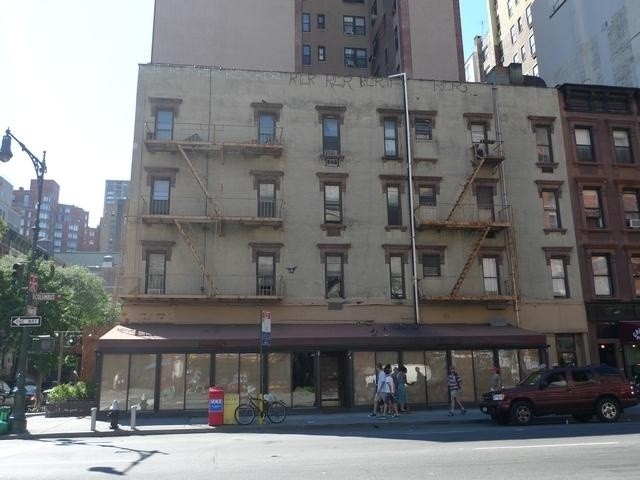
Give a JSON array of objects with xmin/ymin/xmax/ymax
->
[
  {"xmin": 27, "ymin": 291, "xmax": 65, "ymax": 303},
  {"xmin": 11, "ymin": 316, "xmax": 41, "ymax": 327}
]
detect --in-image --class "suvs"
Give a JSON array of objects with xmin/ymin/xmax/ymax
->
[{"xmin": 480, "ymin": 363, "xmax": 640, "ymax": 426}]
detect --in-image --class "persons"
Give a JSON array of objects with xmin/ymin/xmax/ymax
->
[
  {"xmin": 489, "ymin": 367, "xmax": 501, "ymax": 391},
  {"xmin": 446, "ymin": 366, "xmax": 466, "ymax": 416},
  {"xmin": 367, "ymin": 362, "xmax": 411, "ymax": 419},
  {"xmin": 539, "ymin": 363, "xmax": 566, "ymax": 386}
]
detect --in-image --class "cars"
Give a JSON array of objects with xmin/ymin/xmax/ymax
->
[{"xmin": 1, "ymin": 379, "xmax": 54, "ymax": 415}]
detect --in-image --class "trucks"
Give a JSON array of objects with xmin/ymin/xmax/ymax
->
[{"xmin": 365, "ymin": 361, "xmax": 432, "ymax": 387}]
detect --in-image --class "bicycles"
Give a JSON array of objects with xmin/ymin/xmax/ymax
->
[{"xmin": 233, "ymin": 392, "xmax": 287, "ymax": 425}]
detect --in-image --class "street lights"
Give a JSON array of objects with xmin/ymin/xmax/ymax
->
[{"xmin": 0, "ymin": 127, "xmax": 48, "ymax": 434}]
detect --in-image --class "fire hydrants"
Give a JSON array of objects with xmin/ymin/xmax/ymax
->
[{"xmin": 106, "ymin": 399, "xmax": 120, "ymax": 429}]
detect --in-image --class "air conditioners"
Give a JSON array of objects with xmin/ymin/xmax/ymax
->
[{"xmin": 627, "ymin": 219, "xmax": 639, "ymax": 228}]
